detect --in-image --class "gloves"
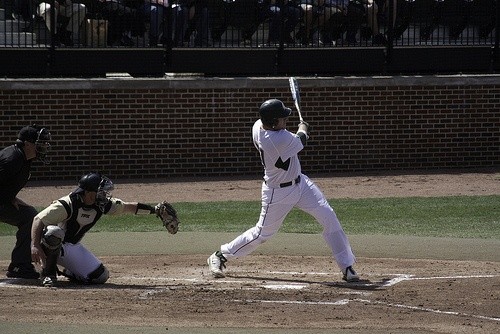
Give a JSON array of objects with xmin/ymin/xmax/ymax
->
[{"xmin": 298, "ymin": 121, "xmax": 309, "ymax": 129}]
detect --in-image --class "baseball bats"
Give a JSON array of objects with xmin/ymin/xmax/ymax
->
[{"xmin": 289, "ymin": 77, "xmax": 309, "ymax": 140}]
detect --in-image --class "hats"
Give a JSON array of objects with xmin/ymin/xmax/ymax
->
[{"xmin": 18, "ymin": 127, "xmax": 38, "ymax": 143}]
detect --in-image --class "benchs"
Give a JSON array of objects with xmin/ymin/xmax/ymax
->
[{"xmin": 0, "ymin": 0, "xmax": 500, "ymax": 45}]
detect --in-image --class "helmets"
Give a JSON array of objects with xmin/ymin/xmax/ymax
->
[
  {"xmin": 74, "ymin": 174, "xmax": 101, "ymax": 194},
  {"xmin": 259, "ymin": 99, "xmax": 292, "ymax": 129}
]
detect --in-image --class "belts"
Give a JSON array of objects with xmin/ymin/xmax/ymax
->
[{"xmin": 265, "ymin": 175, "xmax": 300, "ymax": 188}]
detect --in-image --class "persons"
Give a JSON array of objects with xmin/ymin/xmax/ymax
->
[
  {"xmin": 31, "ymin": 173, "xmax": 181, "ymax": 285},
  {"xmin": 5, "ymin": 0, "xmax": 387, "ymax": 46},
  {"xmin": 206, "ymin": 98, "xmax": 360, "ymax": 280},
  {"xmin": 0, "ymin": 127, "xmax": 52, "ymax": 280}
]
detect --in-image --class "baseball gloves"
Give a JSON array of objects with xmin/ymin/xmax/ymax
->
[{"xmin": 156, "ymin": 200, "xmax": 179, "ymax": 234}]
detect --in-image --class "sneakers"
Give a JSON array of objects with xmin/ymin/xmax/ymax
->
[
  {"xmin": 40, "ymin": 269, "xmax": 57, "ymax": 287},
  {"xmin": 207, "ymin": 251, "xmax": 227, "ymax": 278},
  {"xmin": 342, "ymin": 266, "xmax": 359, "ymax": 282},
  {"xmin": 6, "ymin": 263, "xmax": 40, "ymax": 279}
]
